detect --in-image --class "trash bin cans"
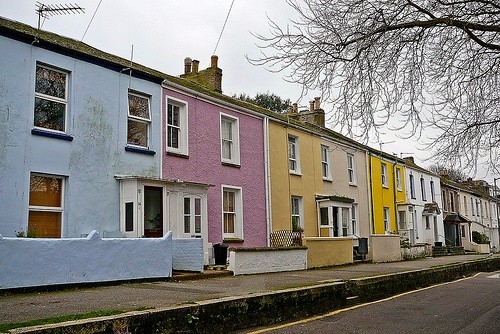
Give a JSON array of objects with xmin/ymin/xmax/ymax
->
[
  {"xmin": 434, "ymin": 242, "xmax": 442, "ymax": 257},
  {"xmin": 213, "ymin": 243, "xmax": 229, "ymax": 265}
]
